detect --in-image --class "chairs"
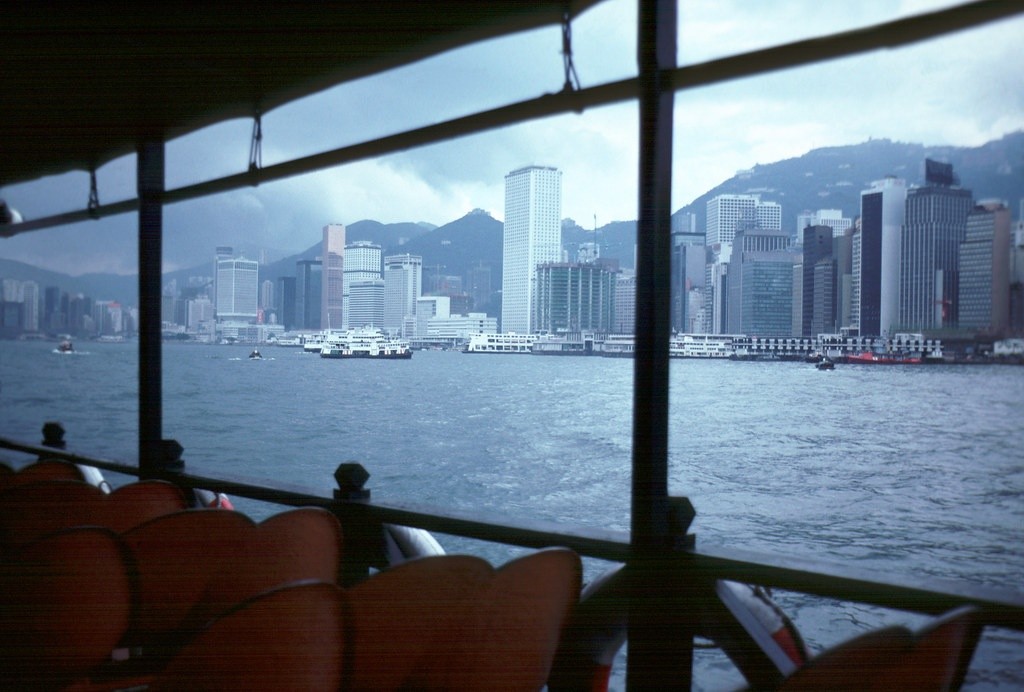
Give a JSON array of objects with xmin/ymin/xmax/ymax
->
[{"xmin": 0, "ymin": 456, "xmax": 989, "ymax": 692}]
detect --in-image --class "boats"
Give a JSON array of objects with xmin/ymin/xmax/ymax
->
[
  {"xmin": 727, "ymin": 350, "xmax": 759, "ymax": 362},
  {"xmin": 849, "ymin": 350, "xmax": 923, "ymax": 365},
  {"xmin": 600, "ymin": 333, "xmax": 733, "ymax": 359},
  {"xmin": 301, "ymin": 312, "xmax": 414, "ymax": 359},
  {"xmin": 816, "ymin": 361, "xmax": 834, "ymax": 370},
  {"xmin": 806, "ymin": 350, "xmax": 826, "ymax": 363},
  {"xmin": 248, "ymin": 349, "xmax": 263, "ymax": 360},
  {"xmin": 460, "ymin": 332, "xmax": 595, "ymax": 355},
  {"xmin": 57, "ymin": 342, "xmax": 73, "ymax": 351},
  {"xmin": 778, "ymin": 352, "xmax": 805, "ymax": 362}
]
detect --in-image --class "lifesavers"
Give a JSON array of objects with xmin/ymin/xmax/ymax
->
[
  {"xmin": 108, "ymin": 487, "xmax": 240, "ymax": 512},
  {"xmin": 19, "ymin": 459, "xmax": 115, "ymax": 497},
  {"xmin": 334, "ymin": 500, "xmax": 447, "ymax": 558},
  {"xmin": 541, "ymin": 560, "xmax": 809, "ymax": 692}
]
